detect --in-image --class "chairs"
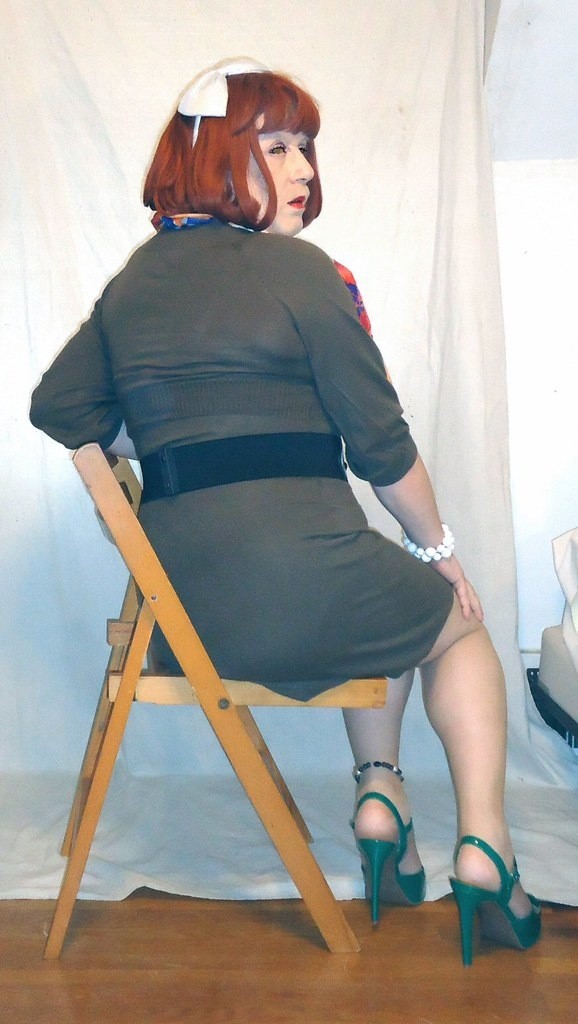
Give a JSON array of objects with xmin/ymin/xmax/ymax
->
[{"xmin": 42, "ymin": 442, "xmax": 389, "ymax": 972}]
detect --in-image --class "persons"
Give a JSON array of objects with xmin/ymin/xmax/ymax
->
[{"xmin": 28, "ymin": 66, "xmax": 545, "ymax": 968}]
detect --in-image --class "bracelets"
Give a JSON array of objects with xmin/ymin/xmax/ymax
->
[{"xmin": 398, "ymin": 518, "xmax": 457, "ymax": 564}]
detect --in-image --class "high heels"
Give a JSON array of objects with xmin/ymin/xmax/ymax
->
[
  {"xmin": 349, "ymin": 791, "xmax": 426, "ymax": 930},
  {"xmin": 449, "ymin": 835, "xmax": 543, "ymax": 966}
]
